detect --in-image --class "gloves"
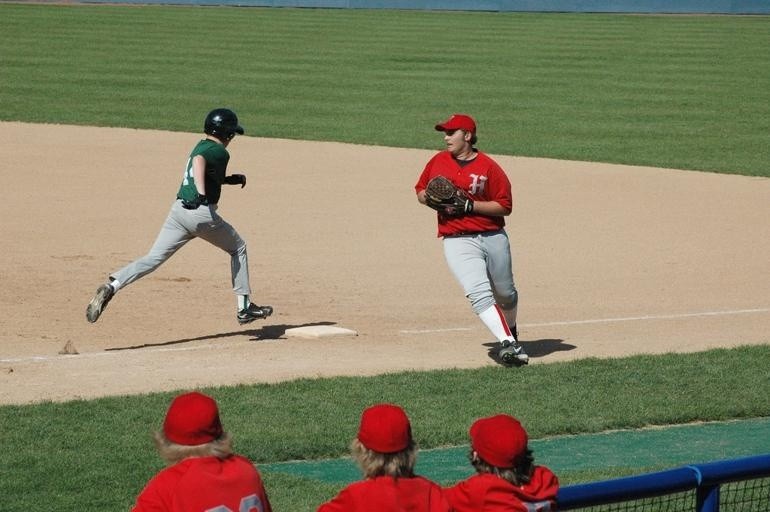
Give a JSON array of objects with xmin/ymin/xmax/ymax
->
[
  {"xmin": 230, "ymin": 173, "xmax": 247, "ymax": 188},
  {"xmin": 182, "ymin": 193, "xmax": 206, "ymax": 210}
]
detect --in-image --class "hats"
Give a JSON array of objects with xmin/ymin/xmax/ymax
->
[
  {"xmin": 469, "ymin": 413, "xmax": 528, "ymax": 470},
  {"xmin": 162, "ymin": 391, "xmax": 222, "ymax": 446},
  {"xmin": 357, "ymin": 402, "xmax": 414, "ymax": 454},
  {"xmin": 435, "ymin": 114, "xmax": 476, "ymax": 134}
]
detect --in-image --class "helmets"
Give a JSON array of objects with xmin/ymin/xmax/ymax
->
[{"xmin": 204, "ymin": 107, "xmax": 244, "ymax": 138}]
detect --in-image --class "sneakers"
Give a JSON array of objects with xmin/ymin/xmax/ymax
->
[
  {"xmin": 497, "ymin": 338, "xmax": 530, "ymax": 364},
  {"xmin": 84, "ymin": 283, "xmax": 115, "ymax": 323},
  {"xmin": 236, "ymin": 302, "xmax": 273, "ymax": 325}
]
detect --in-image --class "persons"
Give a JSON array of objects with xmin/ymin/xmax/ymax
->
[
  {"xmin": 414, "ymin": 113, "xmax": 529, "ymax": 367},
  {"xmin": 316, "ymin": 404, "xmax": 453, "ymax": 512},
  {"xmin": 442, "ymin": 415, "xmax": 558, "ymax": 512},
  {"xmin": 85, "ymin": 107, "xmax": 274, "ymax": 325},
  {"xmin": 130, "ymin": 392, "xmax": 271, "ymax": 511}
]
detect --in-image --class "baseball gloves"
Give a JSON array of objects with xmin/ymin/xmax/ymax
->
[{"xmin": 426, "ymin": 174, "xmax": 473, "ymax": 216}]
